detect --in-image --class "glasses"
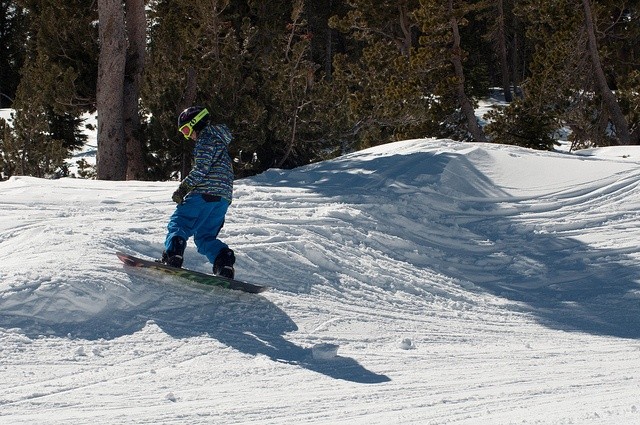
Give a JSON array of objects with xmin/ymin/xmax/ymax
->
[{"xmin": 179, "ymin": 108, "xmax": 209, "ymax": 139}]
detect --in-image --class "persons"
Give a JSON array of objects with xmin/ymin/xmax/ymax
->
[{"xmin": 155, "ymin": 106, "xmax": 235, "ymax": 278}]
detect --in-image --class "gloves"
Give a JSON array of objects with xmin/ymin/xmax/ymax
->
[{"xmin": 173, "ymin": 184, "xmax": 188, "ymax": 204}]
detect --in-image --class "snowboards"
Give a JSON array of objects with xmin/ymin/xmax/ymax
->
[{"xmin": 116, "ymin": 252, "xmax": 265, "ymax": 293}]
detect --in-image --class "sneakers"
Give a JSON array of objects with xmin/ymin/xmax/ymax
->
[
  {"xmin": 157, "ymin": 236, "xmax": 186, "ymax": 268},
  {"xmin": 213, "ymin": 249, "xmax": 235, "ymax": 279}
]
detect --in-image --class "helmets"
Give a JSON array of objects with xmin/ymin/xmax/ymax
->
[{"xmin": 178, "ymin": 105, "xmax": 209, "ymax": 130}]
